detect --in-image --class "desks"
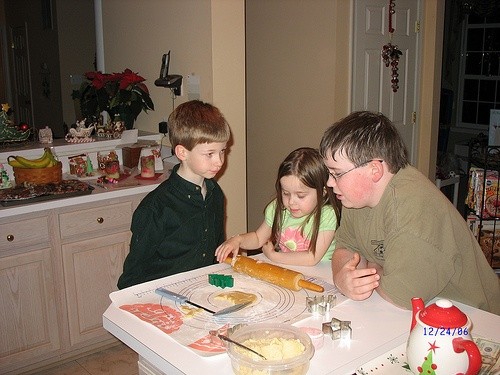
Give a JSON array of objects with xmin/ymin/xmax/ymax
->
[{"xmin": 105, "ymin": 256, "xmax": 500, "ymax": 375}]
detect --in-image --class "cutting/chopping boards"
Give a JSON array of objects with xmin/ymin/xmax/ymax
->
[{"xmin": 118, "ymin": 259, "xmax": 350, "ymax": 357}]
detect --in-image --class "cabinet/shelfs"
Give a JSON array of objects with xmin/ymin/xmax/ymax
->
[
  {"xmin": 0, "ymin": 212, "xmax": 71, "ymax": 375},
  {"xmin": 465, "ymin": 134, "xmax": 500, "ymax": 273},
  {"xmin": 58, "ymin": 191, "xmax": 147, "ymax": 351}
]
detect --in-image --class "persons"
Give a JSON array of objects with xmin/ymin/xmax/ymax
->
[
  {"xmin": 215, "ymin": 147, "xmax": 341, "ymax": 266},
  {"xmin": 318, "ymin": 111, "xmax": 500, "ymax": 316},
  {"xmin": 117, "ymin": 100, "xmax": 231, "ymax": 290}
]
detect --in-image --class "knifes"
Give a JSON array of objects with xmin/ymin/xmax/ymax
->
[{"xmin": 155, "ymin": 286, "xmax": 216, "ymax": 313}]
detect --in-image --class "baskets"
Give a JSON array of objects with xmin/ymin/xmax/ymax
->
[{"xmin": 13, "ymin": 162, "xmax": 62, "ymax": 185}]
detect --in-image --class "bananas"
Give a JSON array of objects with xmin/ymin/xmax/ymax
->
[{"xmin": 8, "ymin": 147, "xmax": 58, "ymax": 168}]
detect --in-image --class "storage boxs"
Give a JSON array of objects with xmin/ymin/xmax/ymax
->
[
  {"xmin": 464, "ymin": 168, "xmax": 500, "ymax": 218},
  {"xmin": 122, "ymin": 147, "xmax": 141, "ymax": 168},
  {"xmin": 466, "ymin": 213, "xmax": 500, "ymax": 269}
]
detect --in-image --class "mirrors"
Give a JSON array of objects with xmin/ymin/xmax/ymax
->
[{"xmin": 0, "ymin": 0, "xmax": 103, "ymax": 139}]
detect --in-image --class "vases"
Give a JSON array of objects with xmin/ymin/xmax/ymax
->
[
  {"xmin": 111, "ymin": 93, "xmax": 142, "ymax": 130},
  {"xmin": 80, "ymin": 93, "xmax": 105, "ymax": 125}
]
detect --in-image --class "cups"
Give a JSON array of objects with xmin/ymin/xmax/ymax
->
[
  {"xmin": 106, "ymin": 161, "xmax": 119, "ymax": 179},
  {"xmin": 141, "ymin": 156, "xmax": 155, "ymax": 178},
  {"xmin": 97, "ymin": 150, "xmax": 118, "ymax": 176}
]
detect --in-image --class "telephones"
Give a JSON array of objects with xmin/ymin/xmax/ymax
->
[{"xmin": 154, "ymin": 49, "xmax": 183, "ymax": 87}]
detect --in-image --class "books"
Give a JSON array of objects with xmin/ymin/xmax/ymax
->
[{"xmin": 465, "ymin": 168, "xmax": 500, "ymax": 268}]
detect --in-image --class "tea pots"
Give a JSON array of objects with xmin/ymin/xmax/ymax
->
[{"xmin": 406, "ymin": 297, "xmax": 482, "ymax": 375}]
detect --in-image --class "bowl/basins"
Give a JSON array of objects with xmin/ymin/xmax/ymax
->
[{"xmin": 226, "ymin": 323, "xmax": 315, "ymax": 375}]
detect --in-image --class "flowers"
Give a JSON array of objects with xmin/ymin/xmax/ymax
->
[
  {"xmin": 107, "ymin": 69, "xmax": 154, "ymax": 123},
  {"xmin": 71, "ymin": 70, "xmax": 109, "ymax": 100}
]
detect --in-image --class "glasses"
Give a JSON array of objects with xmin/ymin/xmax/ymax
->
[{"xmin": 326, "ymin": 160, "xmax": 383, "ymax": 181}]
